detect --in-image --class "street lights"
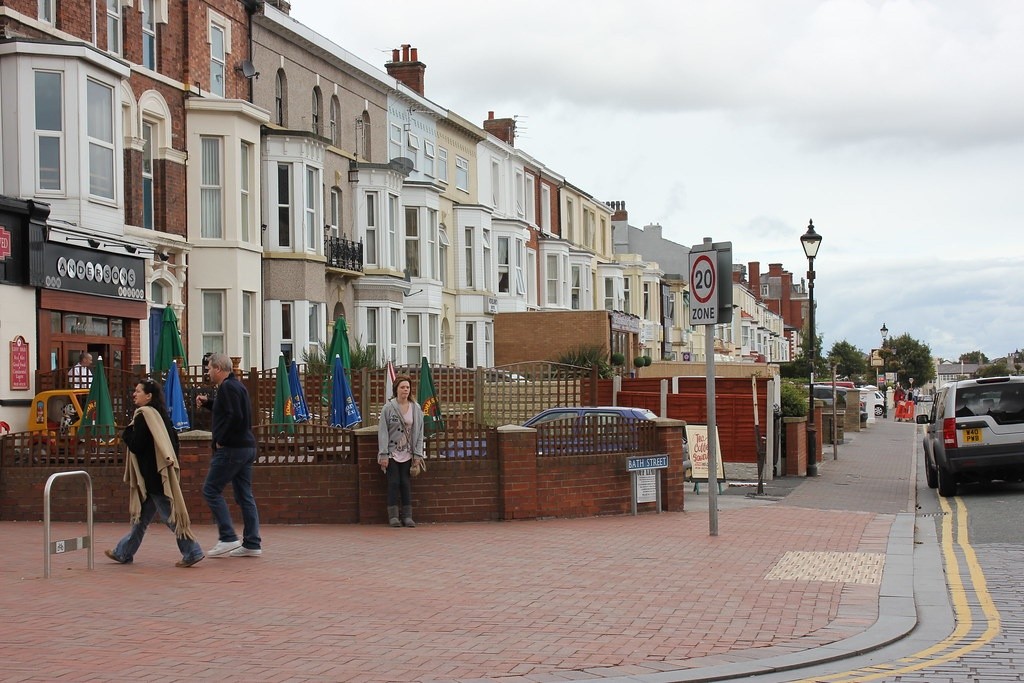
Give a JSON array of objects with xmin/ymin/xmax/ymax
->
[
  {"xmin": 880, "ymin": 323, "xmax": 888, "ymax": 419},
  {"xmin": 891, "ymin": 345, "xmax": 896, "ymax": 408},
  {"xmin": 800, "ymin": 218, "xmax": 822, "ymax": 476}
]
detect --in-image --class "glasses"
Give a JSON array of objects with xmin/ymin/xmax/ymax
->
[{"xmin": 147, "ymin": 378, "xmax": 154, "ymax": 392}]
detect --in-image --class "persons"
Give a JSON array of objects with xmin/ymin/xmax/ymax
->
[
  {"xmin": 202, "ymin": 353, "xmax": 262, "ymax": 557},
  {"xmin": 376, "ymin": 374, "xmax": 424, "ymax": 527},
  {"xmin": 68, "ymin": 352, "xmax": 94, "ymax": 390},
  {"xmin": 104, "ymin": 379, "xmax": 205, "ymax": 568}
]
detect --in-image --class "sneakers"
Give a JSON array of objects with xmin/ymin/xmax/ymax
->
[
  {"xmin": 207, "ymin": 540, "xmax": 241, "ymax": 556},
  {"xmin": 230, "ymin": 546, "xmax": 261, "ymax": 556}
]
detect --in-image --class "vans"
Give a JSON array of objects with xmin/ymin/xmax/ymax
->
[{"xmin": 916, "ymin": 376, "xmax": 1024, "ymax": 496}]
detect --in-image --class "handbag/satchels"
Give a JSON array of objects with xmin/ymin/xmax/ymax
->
[{"xmin": 410, "ymin": 458, "xmax": 420, "ymax": 476}]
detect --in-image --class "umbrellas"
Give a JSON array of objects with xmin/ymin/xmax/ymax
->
[
  {"xmin": 320, "ymin": 313, "xmax": 353, "ymax": 405},
  {"xmin": 420, "ymin": 357, "xmax": 445, "ymax": 460},
  {"xmin": 385, "ymin": 359, "xmax": 396, "ymax": 403},
  {"xmin": 155, "ymin": 301, "xmax": 188, "ymax": 380},
  {"xmin": 287, "ymin": 358, "xmax": 311, "ymax": 426},
  {"xmin": 328, "ymin": 353, "xmax": 362, "ymax": 450},
  {"xmin": 161, "ymin": 359, "xmax": 191, "ymax": 434},
  {"xmin": 270, "ymin": 351, "xmax": 300, "ymax": 442},
  {"xmin": 76, "ymin": 356, "xmax": 116, "ymax": 444}
]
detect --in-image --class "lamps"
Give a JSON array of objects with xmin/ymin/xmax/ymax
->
[
  {"xmin": 104, "ymin": 243, "xmax": 137, "ymax": 253},
  {"xmin": 65, "ymin": 237, "xmax": 101, "ymax": 249},
  {"xmin": 139, "ymin": 252, "xmax": 171, "ymax": 262}
]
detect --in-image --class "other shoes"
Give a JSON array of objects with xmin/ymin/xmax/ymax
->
[
  {"xmin": 105, "ymin": 549, "xmax": 132, "ymax": 563},
  {"xmin": 175, "ymin": 555, "xmax": 204, "ymax": 568}
]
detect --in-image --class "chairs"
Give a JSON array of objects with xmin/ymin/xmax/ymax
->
[{"xmin": 993, "ymin": 389, "xmax": 1024, "ymax": 414}]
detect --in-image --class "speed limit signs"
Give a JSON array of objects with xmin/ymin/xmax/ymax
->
[{"xmin": 691, "ymin": 255, "xmax": 716, "ymax": 303}]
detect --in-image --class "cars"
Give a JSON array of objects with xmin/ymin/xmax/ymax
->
[
  {"xmin": 447, "ymin": 407, "xmax": 691, "ymax": 470},
  {"xmin": 917, "ymin": 395, "xmax": 933, "ymax": 402},
  {"xmin": 26, "ymin": 388, "xmax": 120, "ymax": 462},
  {"xmin": 822, "ymin": 381, "xmax": 864, "ymax": 407},
  {"xmin": 470, "ymin": 367, "xmax": 529, "ymax": 382},
  {"xmin": 805, "ymin": 385, "xmax": 868, "ymax": 422},
  {"xmin": 875, "ymin": 391, "xmax": 885, "ymax": 416}
]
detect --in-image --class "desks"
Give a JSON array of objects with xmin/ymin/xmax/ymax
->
[{"xmin": 260, "ymin": 437, "xmax": 320, "ymax": 454}]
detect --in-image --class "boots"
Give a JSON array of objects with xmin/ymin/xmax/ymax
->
[
  {"xmin": 387, "ymin": 505, "xmax": 401, "ymax": 527},
  {"xmin": 402, "ymin": 505, "xmax": 415, "ymax": 526}
]
